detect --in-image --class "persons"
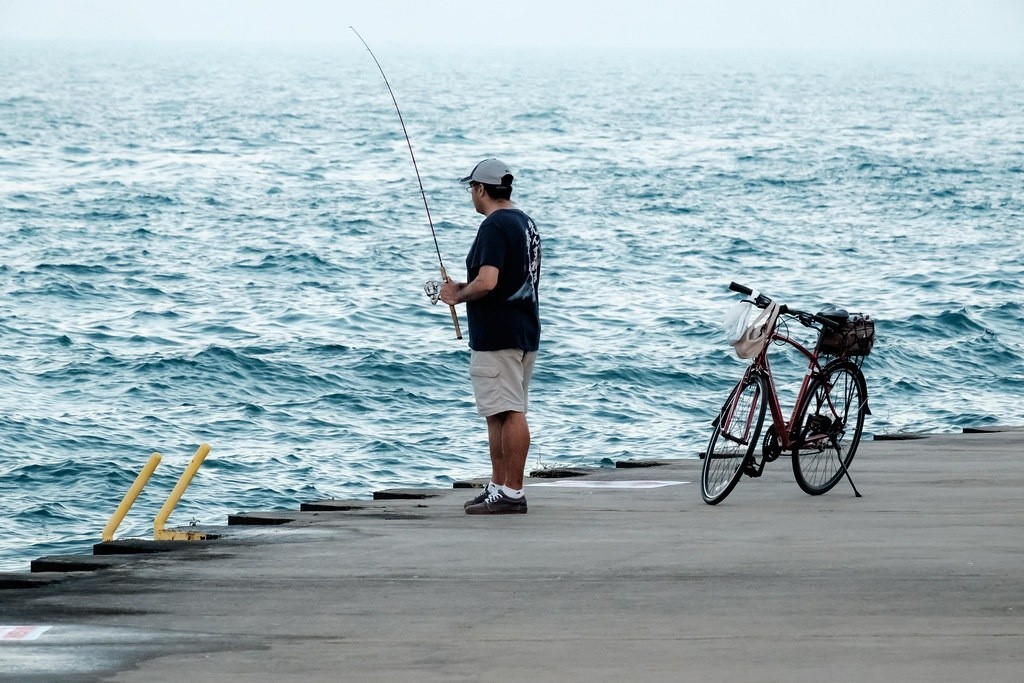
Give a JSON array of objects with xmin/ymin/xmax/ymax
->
[{"xmin": 439, "ymin": 159, "xmax": 541, "ymax": 515}]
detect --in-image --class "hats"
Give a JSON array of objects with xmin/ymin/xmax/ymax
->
[{"xmin": 458, "ymin": 158, "xmax": 514, "ymax": 189}]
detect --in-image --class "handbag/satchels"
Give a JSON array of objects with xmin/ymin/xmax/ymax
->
[
  {"xmin": 734, "ymin": 301, "xmax": 781, "ymax": 359},
  {"xmin": 727, "ymin": 289, "xmax": 761, "ymax": 345}
]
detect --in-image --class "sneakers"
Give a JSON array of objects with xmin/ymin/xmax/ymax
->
[
  {"xmin": 464, "ymin": 485, "xmax": 492, "ymax": 508},
  {"xmin": 465, "ymin": 488, "xmax": 528, "ymax": 514}
]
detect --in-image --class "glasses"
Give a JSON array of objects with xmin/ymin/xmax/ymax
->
[{"xmin": 466, "ymin": 183, "xmax": 480, "ymax": 193}]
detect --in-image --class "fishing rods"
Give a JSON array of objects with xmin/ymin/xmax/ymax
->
[{"xmin": 348, "ymin": 25, "xmax": 462, "ymax": 340}]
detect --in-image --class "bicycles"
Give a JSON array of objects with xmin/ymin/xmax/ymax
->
[{"xmin": 697, "ymin": 280, "xmax": 878, "ymax": 506}]
